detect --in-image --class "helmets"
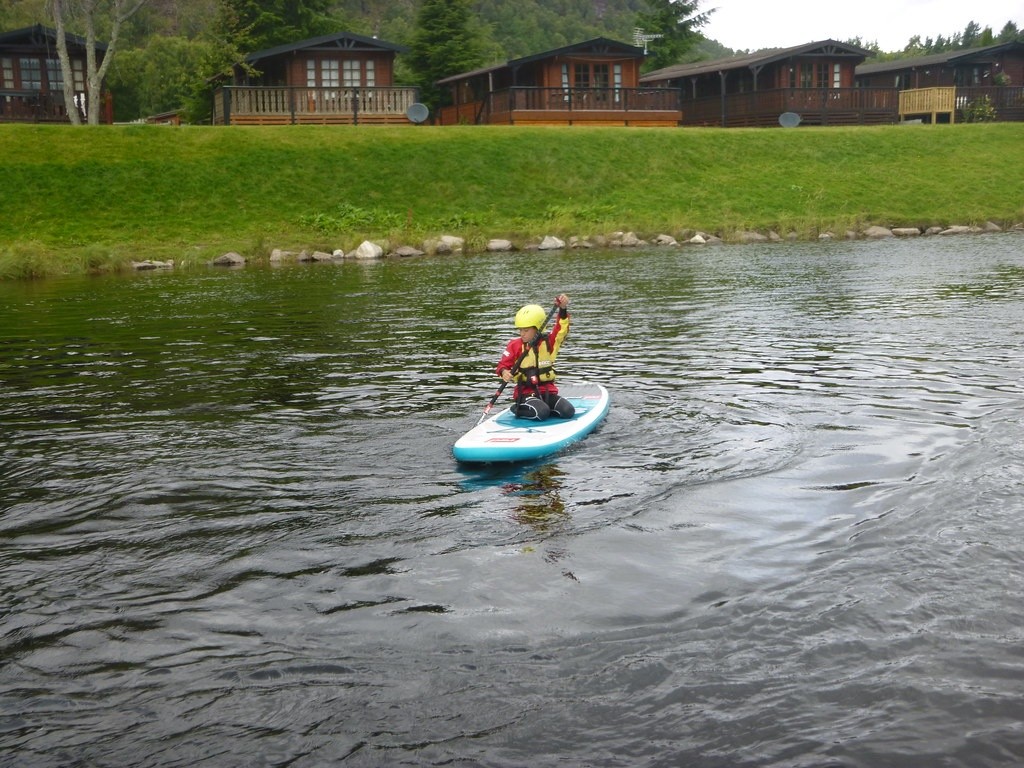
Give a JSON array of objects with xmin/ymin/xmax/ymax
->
[{"xmin": 515, "ymin": 305, "xmax": 547, "ymax": 334}]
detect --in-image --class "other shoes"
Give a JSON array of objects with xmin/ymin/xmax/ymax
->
[{"xmin": 510, "ymin": 404, "xmax": 518, "ymax": 417}]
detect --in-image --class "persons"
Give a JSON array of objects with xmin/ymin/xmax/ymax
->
[{"xmin": 497, "ymin": 293, "xmax": 575, "ymax": 420}]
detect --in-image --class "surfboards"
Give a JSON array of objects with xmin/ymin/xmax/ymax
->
[{"xmin": 450, "ymin": 379, "xmax": 610, "ymax": 464}]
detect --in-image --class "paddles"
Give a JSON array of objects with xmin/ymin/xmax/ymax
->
[{"xmin": 471, "ymin": 295, "xmax": 561, "ymax": 428}]
